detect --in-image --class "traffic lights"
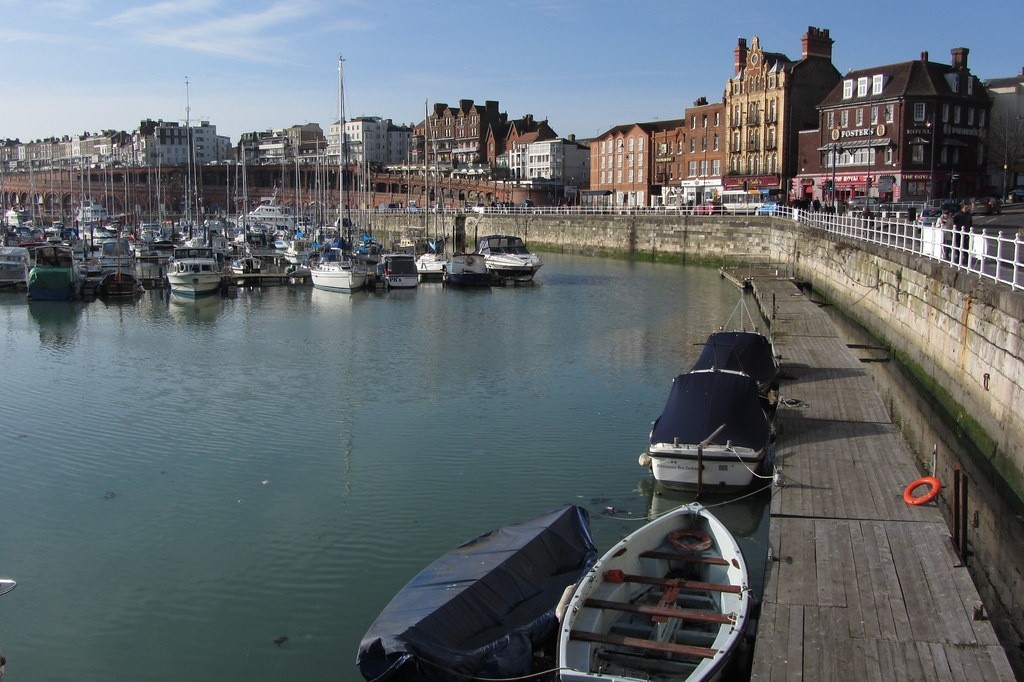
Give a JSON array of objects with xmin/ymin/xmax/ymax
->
[
  {"xmin": 868, "ymin": 178, "xmax": 872, "ymax": 187},
  {"xmin": 828, "ymin": 181, "xmax": 833, "ymax": 190}
]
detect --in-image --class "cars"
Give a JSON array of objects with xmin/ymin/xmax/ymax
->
[
  {"xmin": 691, "ymin": 202, "xmax": 727, "ymax": 216},
  {"xmin": 848, "ymin": 196, "xmax": 892, "ymax": 217},
  {"xmin": 970, "ymin": 197, "xmax": 1001, "ymax": 215},
  {"xmin": 1012, "ymin": 189, "xmax": 1024, "ymax": 198},
  {"xmin": 917, "ymin": 207, "xmax": 943, "ymax": 232},
  {"xmin": 754, "ymin": 201, "xmax": 783, "ymax": 215},
  {"xmin": 401, "ymin": 201, "xmax": 486, "ymax": 214}
]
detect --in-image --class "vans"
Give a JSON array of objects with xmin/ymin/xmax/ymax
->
[
  {"xmin": 220, "ymin": 159, "xmax": 234, "ymax": 165},
  {"xmin": 206, "ymin": 161, "xmax": 218, "ymax": 165}
]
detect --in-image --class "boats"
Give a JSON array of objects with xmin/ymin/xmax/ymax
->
[
  {"xmin": 442, "ymin": 251, "xmax": 491, "ymax": 289},
  {"xmin": 554, "ymin": 498, "xmax": 754, "ymax": 682},
  {"xmin": 640, "ymin": 290, "xmax": 782, "ymax": 494},
  {"xmin": 352, "ymin": 500, "xmax": 599, "ymax": 682},
  {"xmin": 473, "ymin": 235, "xmax": 544, "ymax": 281}
]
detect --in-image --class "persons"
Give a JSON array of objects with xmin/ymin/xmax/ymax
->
[
  {"xmin": 490, "ymin": 200, "xmax": 514, "ymax": 214},
  {"xmin": 861, "ymin": 206, "xmax": 873, "ymax": 239},
  {"xmin": 547, "ymin": 196, "xmax": 574, "ymax": 215},
  {"xmin": 935, "ymin": 203, "xmax": 973, "ymax": 264},
  {"xmin": 794, "ymin": 196, "xmax": 821, "ymax": 214}
]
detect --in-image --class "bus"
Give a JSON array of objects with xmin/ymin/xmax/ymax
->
[{"xmin": 720, "ymin": 189, "xmax": 787, "ymax": 214}]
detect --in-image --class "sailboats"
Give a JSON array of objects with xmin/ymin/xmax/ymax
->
[{"xmin": 0, "ymin": 52, "xmax": 449, "ymax": 299}]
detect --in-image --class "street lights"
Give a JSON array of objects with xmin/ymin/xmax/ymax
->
[
  {"xmin": 925, "ymin": 112, "xmax": 935, "ymax": 204},
  {"xmin": 1002, "ymin": 164, "xmax": 1008, "ymax": 205}
]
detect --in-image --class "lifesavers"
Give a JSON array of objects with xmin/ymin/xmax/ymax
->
[
  {"xmin": 904, "ymin": 477, "xmax": 940, "ymax": 507},
  {"xmin": 179, "ymin": 263, "xmax": 186, "ymax": 273},
  {"xmin": 221, "ymin": 248, "xmax": 229, "ymax": 258},
  {"xmin": 467, "ymin": 257, "xmax": 474, "ymax": 266}
]
